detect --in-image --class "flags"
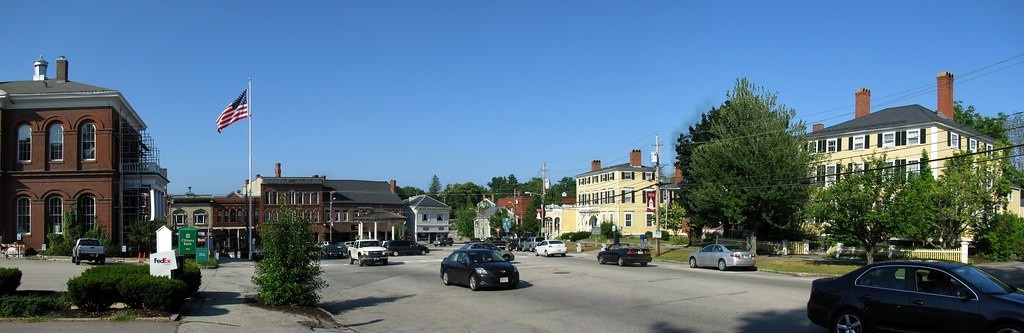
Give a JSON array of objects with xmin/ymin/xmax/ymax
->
[
  {"xmin": 536, "ymin": 208, "xmax": 541, "ymax": 220},
  {"xmin": 216, "ymin": 88, "xmax": 248, "ymax": 133},
  {"xmin": 646, "ymin": 190, "xmax": 655, "ymax": 212}
]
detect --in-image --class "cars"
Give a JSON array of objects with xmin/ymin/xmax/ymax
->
[
  {"xmin": 306, "ymin": 237, "xmax": 356, "ymax": 259},
  {"xmin": 805, "ymin": 258, "xmax": 1024, "ymax": 333},
  {"xmin": 436, "ymin": 248, "xmax": 519, "ymax": 291},
  {"xmin": 432, "ymin": 238, "xmax": 454, "ymax": 247},
  {"xmin": 536, "ymin": 240, "xmax": 568, "ymax": 257},
  {"xmin": 470, "ymin": 235, "xmax": 545, "ymax": 252},
  {"xmin": 378, "ymin": 239, "xmax": 429, "ymax": 256},
  {"xmin": 685, "ymin": 244, "xmax": 757, "ymax": 271},
  {"xmin": 459, "ymin": 242, "xmax": 515, "ymax": 262},
  {"xmin": 596, "ymin": 243, "xmax": 652, "ymax": 268},
  {"xmin": 346, "ymin": 240, "xmax": 388, "ymax": 266}
]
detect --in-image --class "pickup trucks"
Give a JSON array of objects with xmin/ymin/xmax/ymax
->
[{"xmin": 72, "ymin": 237, "xmax": 106, "ymax": 264}]
